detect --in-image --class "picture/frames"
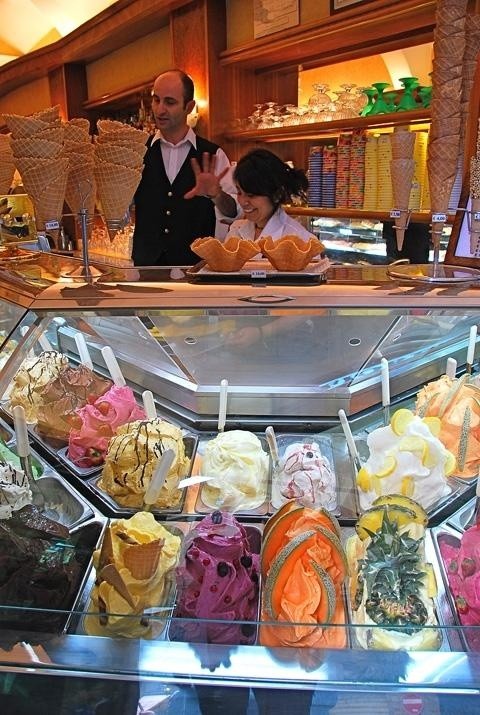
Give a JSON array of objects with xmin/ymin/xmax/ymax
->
[{"xmin": 328, "ymin": 0, "xmax": 377, "ymax": 18}]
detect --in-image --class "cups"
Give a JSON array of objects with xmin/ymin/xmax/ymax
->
[{"xmin": 306, "ymin": 121, "xmax": 431, "ymax": 214}]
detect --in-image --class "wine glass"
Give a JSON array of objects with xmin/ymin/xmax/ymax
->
[{"xmin": 236, "ymin": 84, "xmax": 366, "ymax": 132}]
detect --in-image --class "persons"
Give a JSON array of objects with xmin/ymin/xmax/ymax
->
[
  {"xmin": 223, "ymin": 148, "xmax": 322, "ymax": 260},
  {"xmin": 131, "ymin": 69, "xmax": 244, "ymax": 267}
]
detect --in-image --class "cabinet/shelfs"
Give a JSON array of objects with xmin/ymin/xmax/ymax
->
[
  {"xmin": 1, "ymin": 247, "xmax": 480, "ymax": 714},
  {"xmin": 219, "ymin": 0, "xmax": 436, "ymax": 219}
]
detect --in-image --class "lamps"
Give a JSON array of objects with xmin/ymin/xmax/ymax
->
[{"xmin": 187, "ymin": 103, "xmax": 200, "ymax": 128}]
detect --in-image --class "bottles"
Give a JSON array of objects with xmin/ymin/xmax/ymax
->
[
  {"xmin": 89, "ymin": 107, "xmax": 156, "ymax": 137},
  {"xmin": 357, "ymin": 77, "xmax": 433, "ymax": 116},
  {"xmin": 60, "ymin": 225, "xmax": 75, "ymax": 250}
]
